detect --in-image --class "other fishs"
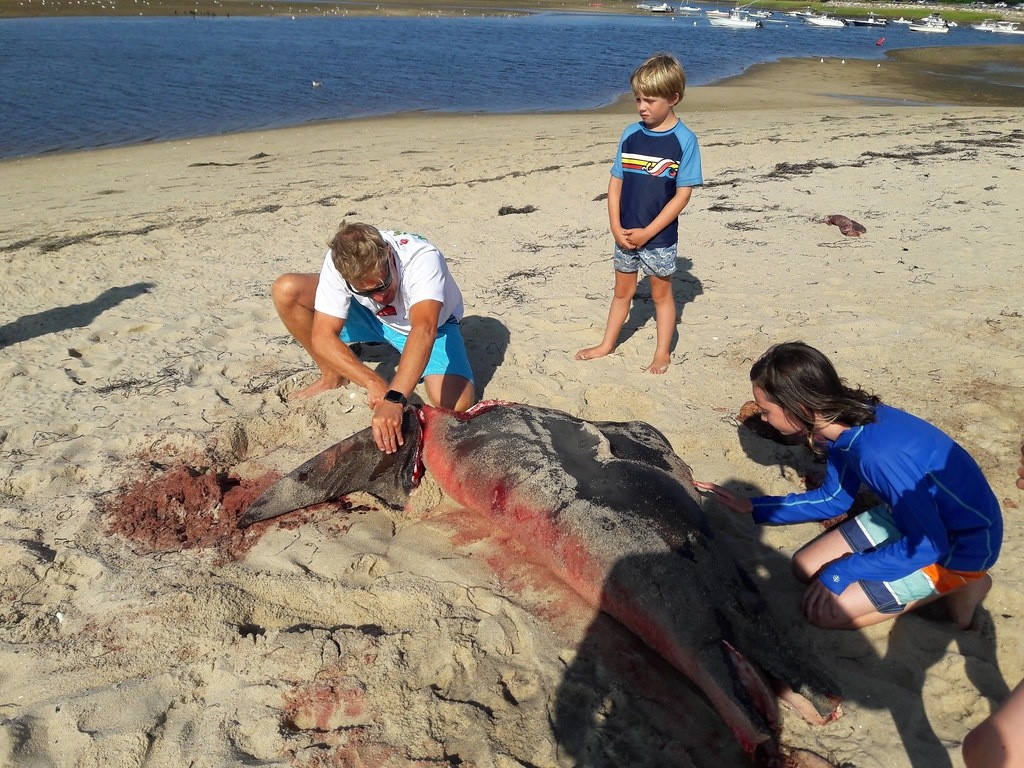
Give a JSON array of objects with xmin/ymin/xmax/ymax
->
[{"xmin": 235, "ymin": 400, "xmax": 846, "ymax": 768}]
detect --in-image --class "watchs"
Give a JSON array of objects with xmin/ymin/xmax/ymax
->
[{"xmin": 384, "ymin": 390, "xmax": 408, "ymax": 409}]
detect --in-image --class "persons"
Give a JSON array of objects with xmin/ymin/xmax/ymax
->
[
  {"xmin": 691, "ymin": 339, "xmax": 1004, "ymax": 630},
  {"xmin": 576, "ymin": 54, "xmax": 704, "ymax": 373},
  {"xmin": 270, "ymin": 222, "xmax": 475, "ymax": 454}
]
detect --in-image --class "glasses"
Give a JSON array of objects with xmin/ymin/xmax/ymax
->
[{"xmin": 345, "ymin": 261, "xmax": 392, "ymax": 300}]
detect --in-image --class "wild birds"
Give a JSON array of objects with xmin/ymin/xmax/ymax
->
[
  {"xmin": 312, "ymin": 80, "xmax": 323, "ymax": 87},
  {"xmin": 876, "ymin": 63, "xmax": 881, "ymax": 69},
  {"xmin": 692, "ymin": 21, "xmax": 697, "ymax": 25},
  {"xmin": 841, "ymin": 59, "xmax": 847, "ymax": 65},
  {"xmin": 670, "ymin": 17, "xmax": 676, "ymax": 21},
  {"xmin": 417, "ymin": 7, "xmax": 530, "ymax": 21},
  {"xmin": 174, "ymin": 0, "xmax": 230, "ymax": 19},
  {"xmin": 375, "ymin": 4, "xmax": 388, "ymax": 17},
  {"xmin": 250, "ymin": 0, "xmax": 350, "ymax": 21},
  {"xmin": 819, "ymin": 57, "xmax": 825, "ymax": 64},
  {"xmin": 20, "ymin": 0, "xmax": 163, "ymax": 16}
]
